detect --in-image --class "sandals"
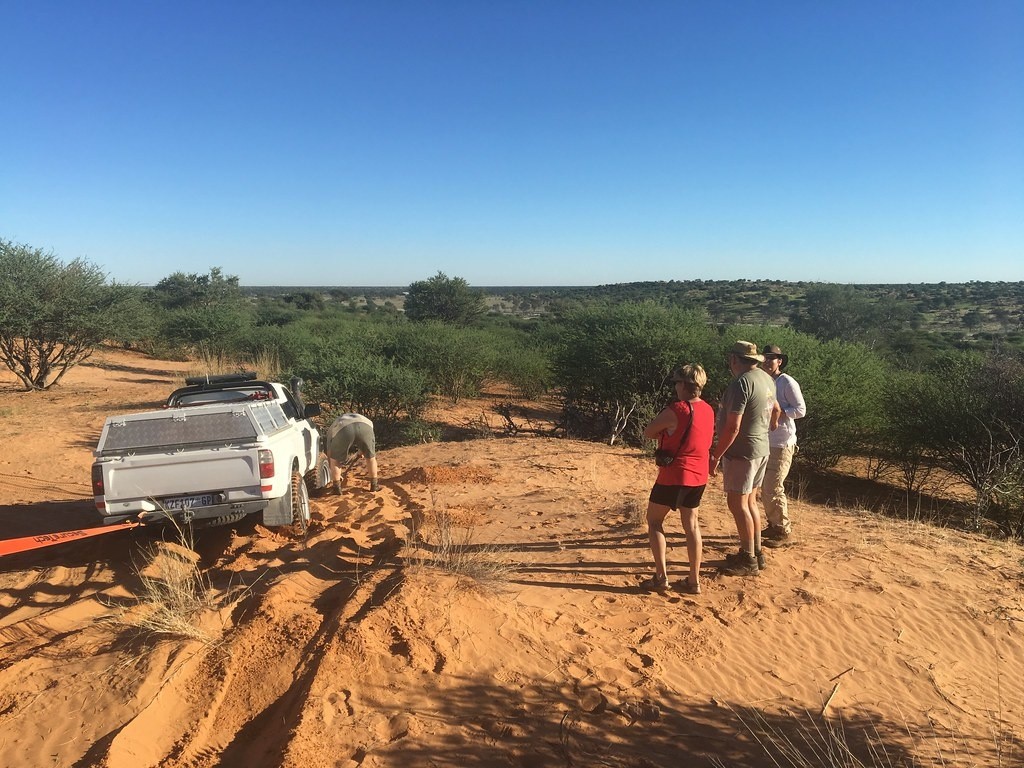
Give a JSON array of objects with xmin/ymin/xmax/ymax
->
[
  {"xmin": 640, "ymin": 575, "xmax": 669, "ymax": 592},
  {"xmin": 676, "ymin": 576, "xmax": 701, "ymax": 594}
]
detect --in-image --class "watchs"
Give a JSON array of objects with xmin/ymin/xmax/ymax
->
[{"xmin": 711, "ymin": 454, "xmax": 719, "ymax": 462}]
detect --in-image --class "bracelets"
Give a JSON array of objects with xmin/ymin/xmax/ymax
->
[{"xmin": 781, "ymin": 409, "xmax": 785, "ymax": 414}]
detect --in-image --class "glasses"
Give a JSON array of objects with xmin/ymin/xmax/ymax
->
[
  {"xmin": 673, "ymin": 380, "xmax": 684, "ymax": 385},
  {"xmin": 767, "ymin": 354, "xmax": 778, "ymax": 360}
]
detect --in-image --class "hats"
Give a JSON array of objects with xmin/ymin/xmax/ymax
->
[
  {"xmin": 724, "ymin": 340, "xmax": 764, "ymax": 362},
  {"xmin": 760, "ymin": 345, "xmax": 788, "ymax": 370}
]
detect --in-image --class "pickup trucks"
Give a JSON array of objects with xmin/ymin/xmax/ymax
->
[{"xmin": 91, "ymin": 371, "xmax": 331, "ymax": 540}]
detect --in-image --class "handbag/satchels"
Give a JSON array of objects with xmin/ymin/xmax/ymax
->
[{"xmin": 655, "ymin": 400, "xmax": 694, "ymax": 465}]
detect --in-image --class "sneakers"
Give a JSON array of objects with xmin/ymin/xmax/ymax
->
[
  {"xmin": 726, "ymin": 548, "xmax": 766, "ymax": 569},
  {"xmin": 723, "ymin": 552, "xmax": 760, "ymax": 575}
]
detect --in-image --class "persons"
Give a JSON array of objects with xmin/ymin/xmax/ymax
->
[
  {"xmin": 760, "ymin": 345, "xmax": 806, "ymax": 547},
  {"xmin": 641, "ymin": 365, "xmax": 714, "ymax": 593},
  {"xmin": 709, "ymin": 340, "xmax": 780, "ymax": 577},
  {"xmin": 326, "ymin": 413, "xmax": 380, "ymax": 495}
]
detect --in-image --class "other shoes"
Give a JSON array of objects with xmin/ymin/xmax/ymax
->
[
  {"xmin": 764, "ymin": 532, "xmax": 789, "ymax": 547},
  {"xmin": 371, "ymin": 485, "xmax": 379, "ymax": 492},
  {"xmin": 332, "ymin": 488, "xmax": 342, "ymax": 495},
  {"xmin": 762, "ymin": 527, "xmax": 773, "ymax": 537}
]
